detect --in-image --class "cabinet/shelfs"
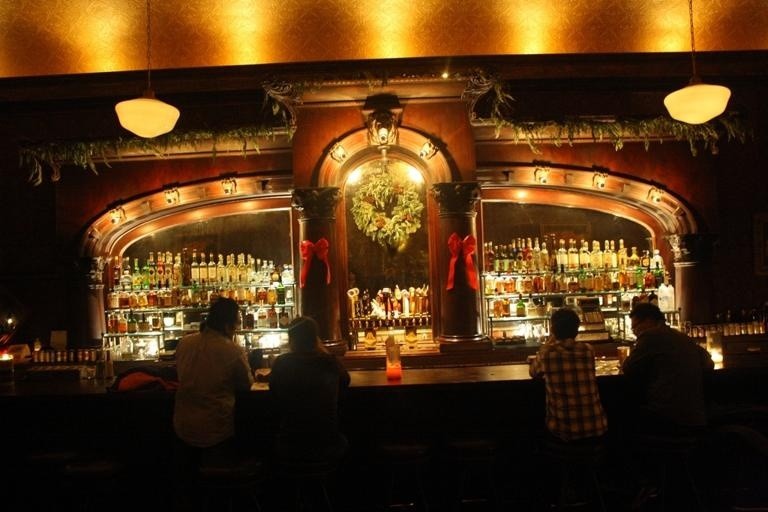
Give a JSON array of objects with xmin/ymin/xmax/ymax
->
[
  {"xmin": 77, "ymin": 179, "xmax": 297, "ymax": 359},
  {"xmin": 318, "ymin": 147, "xmax": 452, "ymax": 354},
  {"xmin": 472, "ymin": 182, "xmax": 680, "ymax": 342}
]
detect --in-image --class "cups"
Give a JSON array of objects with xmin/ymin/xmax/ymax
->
[{"xmin": 386, "ymin": 344, "xmax": 402, "ymax": 381}]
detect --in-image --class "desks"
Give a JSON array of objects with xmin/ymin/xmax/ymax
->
[{"xmin": 1, "ymin": 344, "xmax": 767, "ymax": 442}]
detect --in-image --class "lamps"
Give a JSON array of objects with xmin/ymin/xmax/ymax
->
[
  {"xmin": 113, "ymin": 1, "xmax": 181, "ymax": 137},
  {"xmin": 663, "ymin": 3, "xmax": 730, "ymax": 125}
]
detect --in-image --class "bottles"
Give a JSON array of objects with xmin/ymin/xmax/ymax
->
[
  {"xmin": 494, "ymin": 289, "xmax": 501, "ymax": 319},
  {"xmin": 252, "ymin": 258, "xmax": 280, "ymax": 286},
  {"xmin": 247, "ymin": 279, "xmax": 267, "ymax": 304},
  {"xmin": 484, "ymin": 243, "xmax": 488, "ymax": 270},
  {"xmin": 210, "ymin": 281, "xmax": 217, "ymax": 301},
  {"xmin": 152, "ymin": 313, "xmax": 161, "ymax": 331},
  {"xmin": 543, "ymin": 234, "xmax": 551, "ymax": 270},
  {"xmin": 632, "ymin": 296, "xmax": 640, "ymax": 311},
  {"xmin": 494, "ymin": 246, "xmax": 500, "ymax": 273},
  {"xmin": 717, "ymin": 322, "xmax": 723, "ymax": 337},
  {"xmin": 499, "ymin": 244, "xmax": 505, "ymax": 273},
  {"xmin": 113, "ymin": 312, "xmax": 118, "ymax": 333},
  {"xmin": 594, "ymin": 269, "xmax": 603, "ymax": 291},
  {"xmin": 579, "ymin": 240, "xmax": 591, "ymax": 269},
  {"xmin": 132, "ymin": 258, "xmax": 141, "ymax": 290},
  {"xmin": 505, "ymin": 276, "xmax": 514, "ymax": 294},
  {"xmin": 525, "ymin": 274, "xmax": 533, "ymax": 293},
  {"xmin": 200, "ymin": 253, "xmax": 208, "ymax": 285},
  {"xmin": 267, "ymin": 283, "xmax": 276, "ymax": 305},
  {"xmin": 208, "ymin": 253, "xmax": 217, "ymax": 285},
  {"xmin": 640, "ymin": 292, "xmax": 648, "ymax": 303},
  {"xmin": 516, "ymin": 238, "xmax": 523, "ymax": 273},
  {"xmin": 741, "ymin": 319, "xmax": 748, "ymax": 336},
  {"xmin": 227, "ymin": 275, "xmax": 235, "ymax": 301},
  {"xmin": 121, "ymin": 256, "xmax": 132, "ymax": 291},
  {"xmin": 550, "ymin": 265, "xmax": 561, "ymax": 293},
  {"xmin": 538, "ymin": 297, "xmax": 547, "ymax": 316},
  {"xmin": 517, "ymin": 294, "xmax": 527, "ymax": 317},
  {"xmin": 279, "ymin": 306, "xmax": 289, "ymax": 329},
  {"xmin": 216, "ymin": 254, "xmax": 226, "ymax": 284},
  {"xmin": 142, "ymin": 259, "xmax": 149, "ymax": 290},
  {"xmin": 119, "ymin": 284, "xmax": 130, "ymax": 308},
  {"xmin": 525, "ymin": 238, "xmax": 536, "ymax": 274},
  {"xmin": 759, "ymin": 319, "xmax": 766, "ymax": 334},
  {"xmin": 692, "ymin": 323, "xmax": 698, "ymax": 338},
  {"xmin": 618, "ymin": 240, "xmax": 628, "ymax": 268},
  {"xmin": 734, "ymin": 319, "xmax": 740, "ymax": 336},
  {"xmin": 591, "ymin": 241, "xmax": 602, "ymax": 268},
  {"xmin": 627, "ymin": 263, "xmax": 637, "ymax": 290},
  {"xmin": 113, "ymin": 256, "xmax": 120, "ymax": 290},
  {"xmin": 723, "ymin": 320, "xmax": 729, "ymax": 336},
  {"xmin": 511, "ymin": 239, "xmax": 516, "ymax": 270},
  {"xmin": 650, "ymin": 271, "xmax": 655, "ymax": 289},
  {"xmin": 729, "ymin": 320, "xmax": 735, "ymax": 336},
  {"xmin": 704, "ymin": 323, "xmax": 710, "ymax": 336},
  {"xmin": 655, "ymin": 262, "xmax": 662, "ymax": 288},
  {"xmin": 645, "ymin": 267, "xmax": 650, "ymax": 290},
  {"xmin": 156, "ymin": 252, "xmax": 166, "ymax": 290},
  {"xmin": 649, "ymin": 291, "xmax": 658, "ymax": 307},
  {"xmin": 622, "ymin": 286, "xmax": 630, "ymax": 312},
  {"xmin": 636, "ymin": 267, "xmax": 644, "ymax": 292},
  {"xmin": 612, "ymin": 270, "xmax": 620, "ymax": 290},
  {"xmin": 192, "ymin": 280, "xmax": 199, "ymax": 307},
  {"xmin": 182, "ymin": 288, "xmax": 191, "ymax": 308},
  {"xmin": 237, "ymin": 253, "xmax": 246, "ymax": 284},
  {"xmin": 148, "ymin": 252, "xmax": 156, "ymax": 290},
  {"xmin": 709, "ymin": 322, "xmax": 716, "ymax": 332},
  {"xmin": 138, "ymin": 312, "xmax": 150, "ymax": 332},
  {"xmin": 502, "ymin": 246, "xmax": 509, "ymax": 272},
  {"xmin": 245, "ymin": 301, "xmax": 254, "ymax": 329},
  {"xmin": 485, "ymin": 274, "xmax": 495, "ymax": 296},
  {"xmin": 534, "ymin": 237, "xmax": 541, "ymax": 273},
  {"xmin": 164, "ymin": 279, "xmax": 171, "ymax": 308},
  {"xmin": 665, "ymin": 269, "xmax": 669, "ymax": 285},
  {"xmin": 277, "ymin": 279, "xmax": 285, "ymax": 304},
  {"xmin": 516, "ymin": 275, "xmax": 524, "ymax": 293},
  {"xmin": 200, "ymin": 279, "xmax": 208, "ymax": 307},
  {"xmin": 495, "ymin": 275, "xmax": 504, "ymax": 296},
  {"xmin": 653, "ymin": 249, "xmax": 663, "ymax": 267},
  {"xmin": 604, "ymin": 240, "xmax": 612, "ymax": 270},
  {"xmin": 105, "ymin": 350, "xmax": 113, "ymax": 379},
  {"xmin": 508, "ymin": 244, "xmax": 516, "ymax": 271},
  {"xmin": 269, "ymin": 305, "xmax": 277, "ymax": 329},
  {"xmin": 107, "ymin": 312, "xmax": 113, "ymax": 334},
  {"xmin": 107, "ymin": 287, "xmax": 119, "ymax": 310},
  {"xmin": 660, "ymin": 270, "xmax": 664, "ymax": 283},
  {"xmin": 578, "ymin": 265, "xmax": 587, "ymax": 292},
  {"xmin": 697, "ymin": 323, "xmax": 705, "ymax": 338},
  {"xmin": 586, "ymin": 263, "xmax": 594, "ymax": 291},
  {"xmin": 502, "ymin": 297, "xmax": 510, "ymax": 317},
  {"xmin": 603, "ymin": 263, "xmax": 613, "ymax": 290},
  {"xmin": 658, "ymin": 276, "xmax": 668, "ymax": 312},
  {"xmin": 559, "ymin": 264, "xmax": 568, "ymax": 292},
  {"xmin": 218, "ymin": 276, "xmax": 228, "ymax": 299},
  {"xmin": 226, "ymin": 253, "xmax": 237, "ymax": 285},
  {"xmin": 568, "ymin": 274, "xmax": 576, "ymax": 291},
  {"xmin": 257, "ymin": 300, "xmax": 267, "ymax": 328},
  {"xmin": 32, "ymin": 349, "xmax": 96, "ymax": 367},
  {"xmin": 713, "ymin": 303, "xmax": 763, "ymax": 322},
  {"xmin": 533, "ymin": 268, "xmax": 544, "ymax": 294},
  {"xmin": 171, "ymin": 287, "xmax": 177, "ymax": 306},
  {"xmin": 630, "ymin": 247, "xmax": 640, "ymax": 267},
  {"xmin": 286, "ymin": 287, "xmax": 292, "ymax": 305},
  {"xmin": 550, "ymin": 233, "xmax": 559, "ymax": 273},
  {"xmin": 619, "ymin": 265, "xmax": 628, "ymax": 293},
  {"xmin": 157, "ymin": 281, "xmax": 164, "ymax": 308},
  {"xmin": 640, "ymin": 249, "xmax": 651, "ymax": 268},
  {"xmin": 182, "ymin": 248, "xmax": 192, "ymax": 287},
  {"xmin": 521, "ymin": 238, "xmax": 527, "ymax": 274},
  {"xmin": 138, "ymin": 281, "xmax": 148, "ymax": 307},
  {"xmin": 191, "ymin": 248, "xmax": 199, "ymax": 285},
  {"xmin": 527, "ymin": 293, "xmax": 536, "ymax": 316},
  {"xmin": 282, "ymin": 264, "xmax": 292, "ymax": 286},
  {"xmin": 747, "ymin": 320, "xmax": 754, "ymax": 336},
  {"xmin": 668, "ymin": 279, "xmax": 674, "ymax": 312},
  {"xmin": 127, "ymin": 310, "xmax": 137, "ymax": 334},
  {"xmin": 119, "ymin": 311, "xmax": 127, "ymax": 333},
  {"xmin": 752, "ymin": 319, "xmax": 760, "ymax": 335},
  {"xmin": 96, "ymin": 350, "xmax": 104, "ymax": 379},
  {"xmin": 610, "ymin": 240, "xmax": 618, "ymax": 268},
  {"xmin": 385, "ymin": 327, "xmax": 402, "ymax": 382},
  {"xmin": 541, "ymin": 242, "xmax": 549, "ymax": 274},
  {"xmin": 568, "ymin": 239, "xmax": 579, "ymax": 271},
  {"xmin": 176, "ymin": 286, "xmax": 180, "ymax": 306},
  {"xmin": 247, "ymin": 254, "xmax": 252, "ymax": 284},
  {"xmin": 148, "ymin": 283, "xmax": 157, "ymax": 306},
  {"xmin": 162, "ymin": 251, "xmax": 181, "ymax": 287},
  {"xmin": 129, "ymin": 282, "xmax": 138, "ymax": 307},
  {"xmin": 237, "ymin": 275, "xmax": 246, "ymax": 304},
  {"xmin": 763, "ymin": 302, "xmax": 768, "ymax": 334},
  {"xmin": 544, "ymin": 264, "xmax": 552, "ymax": 293},
  {"xmin": 558, "ymin": 239, "xmax": 569, "ymax": 273},
  {"xmin": 488, "ymin": 241, "xmax": 494, "ymax": 271}
]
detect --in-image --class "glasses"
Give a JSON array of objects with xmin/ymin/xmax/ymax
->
[{"xmin": 631, "ymin": 320, "xmax": 643, "ymax": 331}]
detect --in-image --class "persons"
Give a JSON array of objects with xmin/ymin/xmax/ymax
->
[
  {"xmin": 171, "ymin": 297, "xmax": 255, "ymax": 459},
  {"xmin": 268, "ymin": 316, "xmax": 351, "ymax": 461},
  {"xmin": 528, "ymin": 307, "xmax": 610, "ymax": 454},
  {"xmin": 622, "ymin": 302, "xmax": 715, "ymax": 451}
]
